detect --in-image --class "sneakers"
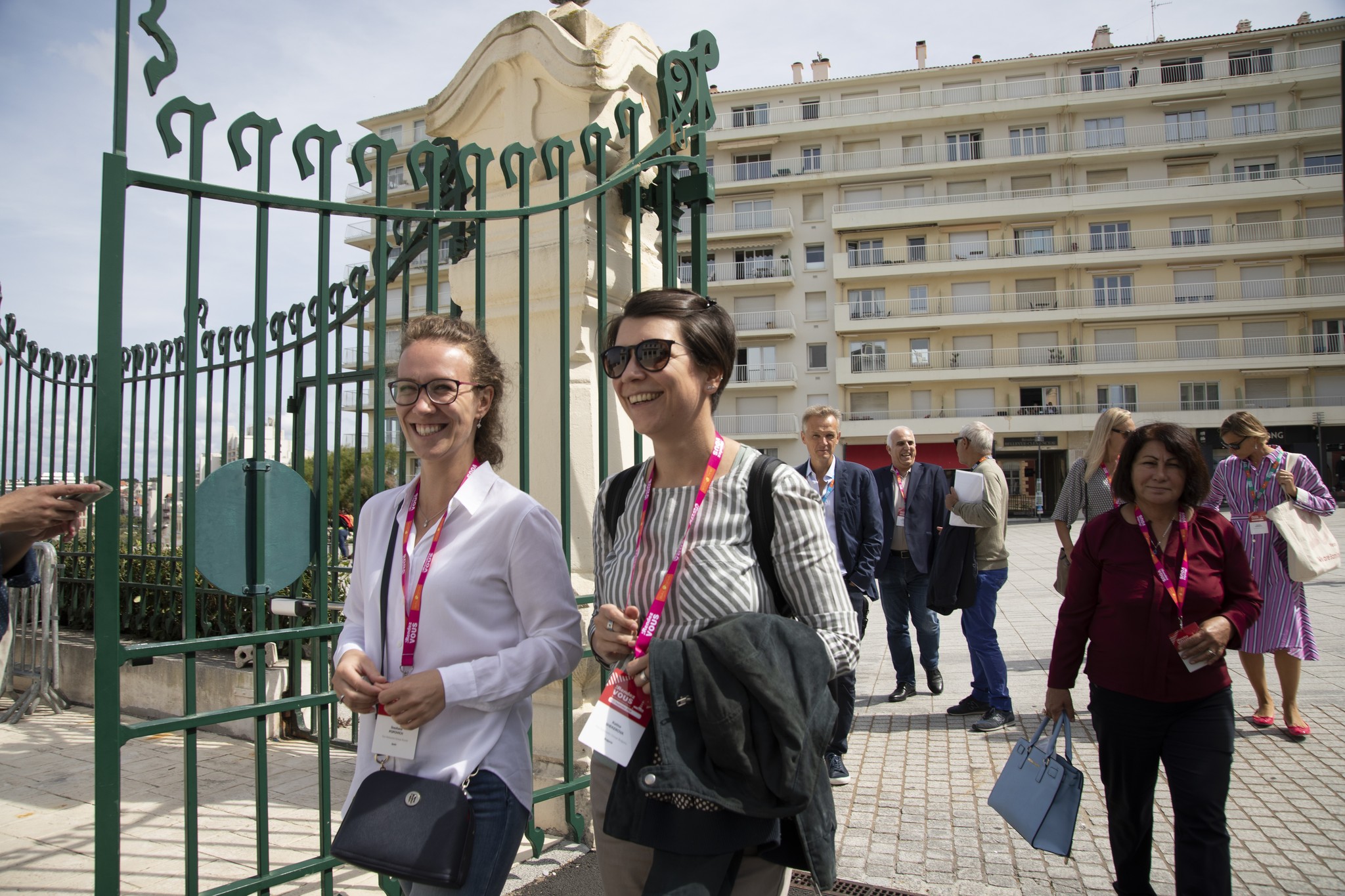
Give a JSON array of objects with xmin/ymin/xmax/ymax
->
[
  {"xmin": 971, "ymin": 705, "xmax": 1016, "ymax": 732},
  {"xmin": 825, "ymin": 753, "xmax": 851, "ymax": 785},
  {"xmin": 947, "ymin": 695, "xmax": 990, "ymax": 716}
]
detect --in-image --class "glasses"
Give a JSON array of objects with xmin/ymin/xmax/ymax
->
[
  {"xmin": 1111, "ymin": 428, "xmax": 1136, "ymax": 439},
  {"xmin": 600, "ymin": 338, "xmax": 695, "ymax": 379},
  {"xmin": 1221, "ymin": 435, "xmax": 1247, "ymax": 450},
  {"xmin": 388, "ymin": 378, "xmax": 487, "ymax": 406},
  {"xmin": 954, "ymin": 436, "xmax": 972, "ymax": 447}
]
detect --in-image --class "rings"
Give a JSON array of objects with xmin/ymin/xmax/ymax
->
[
  {"xmin": 339, "ymin": 695, "xmax": 345, "ymax": 704},
  {"xmin": 1207, "ymin": 649, "xmax": 1219, "ymax": 657},
  {"xmin": 607, "ymin": 620, "xmax": 615, "ymax": 632}
]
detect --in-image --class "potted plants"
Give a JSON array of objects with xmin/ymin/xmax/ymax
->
[
  {"xmin": 1047, "ymin": 348, "xmax": 1064, "ymax": 364},
  {"xmin": 950, "ymin": 353, "xmax": 960, "ymax": 368},
  {"xmin": 780, "ymin": 255, "xmax": 789, "ymax": 276}
]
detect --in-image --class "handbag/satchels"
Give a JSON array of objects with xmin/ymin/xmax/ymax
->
[
  {"xmin": 1053, "ymin": 548, "xmax": 1072, "ymax": 597},
  {"xmin": 329, "ymin": 769, "xmax": 477, "ymax": 890},
  {"xmin": 1265, "ymin": 452, "xmax": 1341, "ymax": 582},
  {"xmin": 987, "ymin": 709, "xmax": 1086, "ymax": 866}
]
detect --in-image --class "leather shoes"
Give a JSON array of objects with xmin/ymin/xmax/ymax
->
[
  {"xmin": 921, "ymin": 664, "xmax": 943, "ymax": 694},
  {"xmin": 888, "ymin": 681, "xmax": 917, "ymax": 701},
  {"xmin": 1283, "ymin": 714, "xmax": 1310, "ymax": 735},
  {"xmin": 1252, "ymin": 707, "xmax": 1274, "ymax": 726}
]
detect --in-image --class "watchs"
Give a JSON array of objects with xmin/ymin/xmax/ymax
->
[{"xmin": 952, "ymin": 501, "xmax": 958, "ymax": 514}]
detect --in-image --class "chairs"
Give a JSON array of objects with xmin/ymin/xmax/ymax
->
[
  {"xmin": 862, "ymin": 416, "xmax": 870, "ymax": 420},
  {"xmin": 1030, "ymin": 302, "xmax": 1040, "ymax": 311},
  {"xmin": 795, "ymin": 166, "xmax": 804, "ymax": 175},
  {"xmin": 707, "ymin": 271, "xmax": 714, "ymax": 281},
  {"xmin": 990, "ymin": 252, "xmax": 1000, "ymax": 259},
  {"xmin": 955, "ymin": 254, "xmax": 967, "ymax": 259},
  {"xmin": 852, "ymin": 417, "xmax": 859, "ymax": 420},
  {"xmin": 1048, "ymin": 300, "xmax": 1058, "ymax": 310}
]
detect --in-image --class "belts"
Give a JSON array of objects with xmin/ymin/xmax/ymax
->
[{"xmin": 889, "ymin": 549, "xmax": 912, "ymax": 559}]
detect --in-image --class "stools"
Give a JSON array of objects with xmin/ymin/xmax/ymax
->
[{"xmin": 772, "ymin": 174, "xmax": 779, "ymax": 177}]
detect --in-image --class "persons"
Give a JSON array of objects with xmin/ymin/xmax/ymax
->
[
  {"xmin": 1197, "ymin": 412, "xmax": 1338, "ymax": 734},
  {"xmin": 1129, "ymin": 67, "xmax": 1139, "ymax": 88},
  {"xmin": 1017, "ymin": 402, "xmax": 1058, "ymax": 415},
  {"xmin": 580, "ymin": 288, "xmax": 864, "ymax": 895},
  {"xmin": 1045, "ymin": 423, "xmax": 1265, "ymax": 896},
  {"xmin": 788, "ymin": 405, "xmax": 883, "ymax": 786},
  {"xmin": 937, "ymin": 421, "xmax": 1016, "ymax": 733},
  {"xmin": 1050, "ymin": 407, "xmax": 1136, "ymax": 563},
  {"xmin": 870, "ymin": 426, "xmax": 951, "ymax": 702},
  {"xmin": 327, "ymin": 317, "xmax": 587, "ymax": 896},
  {"xmin": 4, "ymin": 484, "xmax": 101, "ymax": 693}
]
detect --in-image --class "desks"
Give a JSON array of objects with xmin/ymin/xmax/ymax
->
[
  {"xmin": 1035, "ymin": 303, "xmax": 1050, "ymax": 311},
  {"xmin": 777, "ymin": 169, "xmax": 790, "ymax": 176},
  {"xmin": 970, "ymin": 251, "xmax": 983, "ymax": 258},
  {"xmin": 849, "ymin": 418, "xmax": 873, "ymax": 420}
]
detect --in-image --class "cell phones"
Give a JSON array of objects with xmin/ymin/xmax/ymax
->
[{"xmin": 62, "ymin": 480, "xmax": 114, "ymax": 506}]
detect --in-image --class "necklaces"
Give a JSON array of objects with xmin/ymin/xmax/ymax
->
[
  {"xmin": 1149, "ymin": 504, "xmax": 1178, "ymax": 554},
  {"xmin": 417, "ymin": 502, "xmax": 449, "ymax": 527},
  {"xmin": 1253, "ymin": 460, "xmax": 1258, "ymax": 464}
]
